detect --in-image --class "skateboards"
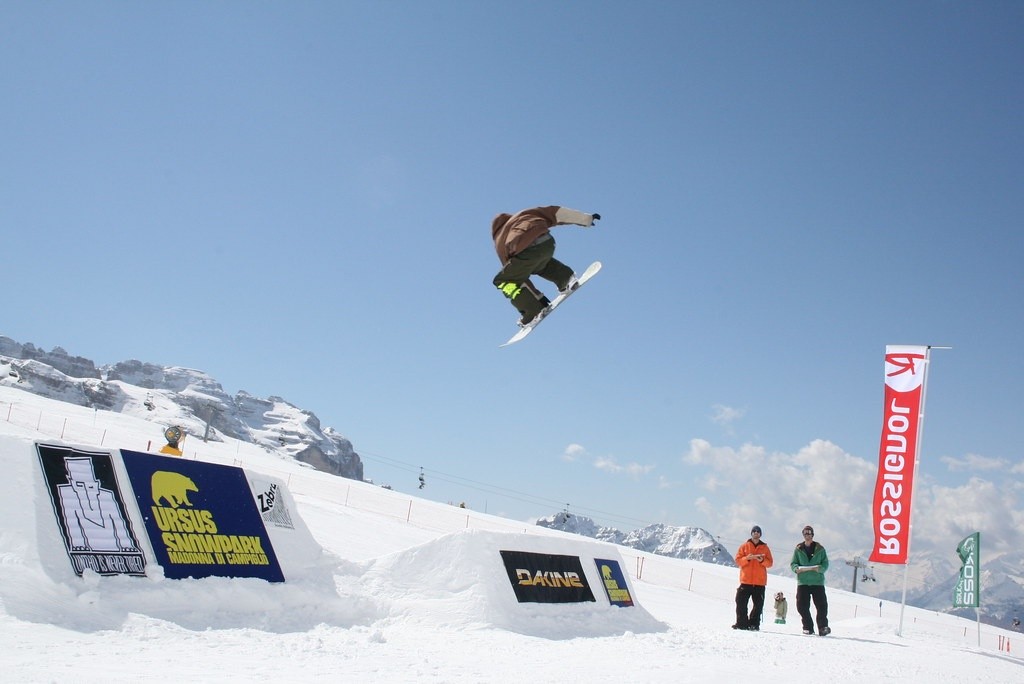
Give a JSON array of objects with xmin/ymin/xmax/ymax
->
[{"xmin": 498, "ymin": 262, "xmax": 602, "ymax": 349}]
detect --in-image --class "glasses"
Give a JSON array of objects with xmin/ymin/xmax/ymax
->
[
  {"xmin": 803, "ymin": 529, "xmax": 812, "ymax": 535},
  {"xmin": 753, "ymin": 530, "xmax": 760, "ymax": 533}
]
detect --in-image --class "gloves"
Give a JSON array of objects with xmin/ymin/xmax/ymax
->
[
  {"xmin": 591, "ymin": 213, "xmax": 602, "ymax": 227},
  {"xmin": 539, "ymin": 296, "xmax": 550, "ymax": 307}
]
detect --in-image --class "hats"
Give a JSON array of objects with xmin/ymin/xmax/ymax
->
[
  {"xmin": 802, "ymin": 526, "xmax": 813, "ymax": 533},
  {"xmin": 751, "ymin": 526, "xmax": 762, "ymax": 536}
]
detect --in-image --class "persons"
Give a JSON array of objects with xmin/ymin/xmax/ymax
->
[
  {"xmin": 773, "ymin": 592, "xmax": 787, "ymax": 623},
  {"xmin": 491, "ymin": 205, "xmax": 600, "ymax": 329},
  {"xmin": 1013, "ymin": 619, "xmax": 1021, "ymax": 628},
  {"xmin": 732, "ymin": 526, "xmax": 772, "ymax": 633},
  {"xmin": 790, "ymin": 526, "xmax": 831, "ymax": 637}
]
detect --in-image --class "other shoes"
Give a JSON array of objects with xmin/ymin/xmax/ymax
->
[
  {"xmin": 802, "ymin": 630, "xmax": 815, "ymax": 636},
  {"xmin": 749, "ymin": 625, "xmax": 759, "ymax": 631},
  {"xmin": 557, "ymin": 271, "xmax": 579, "ymax": 292},
  {"xmin": 517, "ymin": 319, "xmax": 523, "ymax": 327},
  {"xmin": 819, "ymin": 627, "xmax": 831, "ymax": 636},
  {"xmin": 732, "ymin": 623, "xmax": 748, "ymax": 629}
]
